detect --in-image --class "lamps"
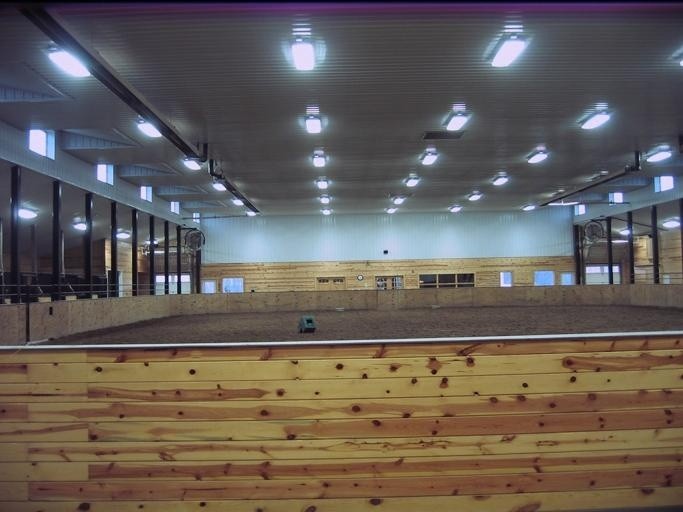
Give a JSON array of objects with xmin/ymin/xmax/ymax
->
[
  {"xmin": 30, "ymin": 129, "xmax": 56, "ymax": 161},
  {"xmin": 94, "ymin": 162, "xmax": 116, "ymax": 186},
  {"xmin": 573, "ymin": 175, "xmax": 674, "ymax": 216},
  {"xmin": 139, "ymin": 186, "xmax": 201, "ymax": 225}
]
